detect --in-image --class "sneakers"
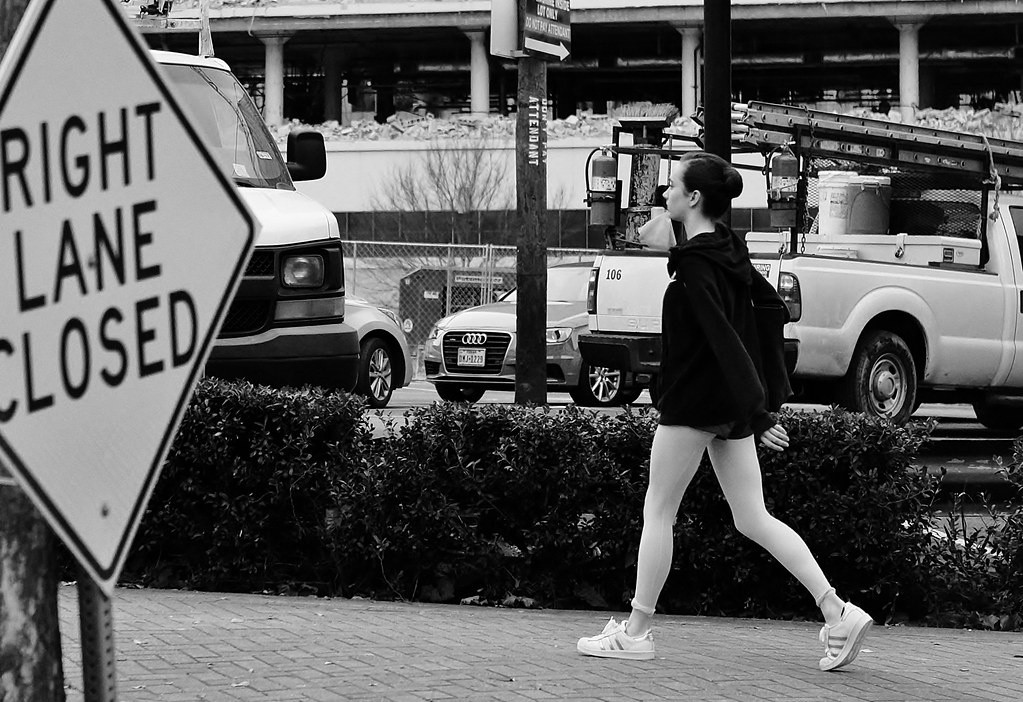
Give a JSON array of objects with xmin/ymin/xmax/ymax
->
[
  {"xmin": 820, "ymin": 602, "xmax": 872, "ymax": 669},
  {"xmin": 575, "ymin": 616, "xmax": 658, "ymax": 661}
]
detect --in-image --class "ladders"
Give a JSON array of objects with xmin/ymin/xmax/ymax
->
[{"xmin": 690, "ymin": 101, "xmax": 1022, "ymax": 184}]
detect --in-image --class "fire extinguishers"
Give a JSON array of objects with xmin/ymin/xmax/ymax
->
[
  {"xmin": 766, "ymin": 140, "xmax": 798, "ymax": 227},
  {"xmin": 583, "ymin": 143, "xmax": 618, "ymax": 226}
]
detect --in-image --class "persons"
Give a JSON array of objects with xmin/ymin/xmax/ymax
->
[{"xmin": 576, "ymin": 151, "xmax": 874, "ymax": 672}]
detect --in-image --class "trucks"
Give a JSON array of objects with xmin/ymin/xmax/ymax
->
[{"xmin": 148, "ymin": 48, "xmax": 361, "ymax": 397}]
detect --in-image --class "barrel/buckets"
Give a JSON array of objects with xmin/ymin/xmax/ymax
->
[{"xmin": 816, "ymin": 170, "xmax": 891, "ymax": 235}]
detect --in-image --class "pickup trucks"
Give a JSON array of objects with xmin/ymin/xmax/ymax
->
[{"xmin": 578, "ymin": 188, "xmax": 1023, "ymax": 432}]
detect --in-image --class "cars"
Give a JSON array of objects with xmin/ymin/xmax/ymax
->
[
  {"xmin": 342, "ymin": 291, "xmax": 413, "ymax": 409},
  {"xmin": 425, "ymin": 261, "xmax": 649, "ymax": 408}
]
